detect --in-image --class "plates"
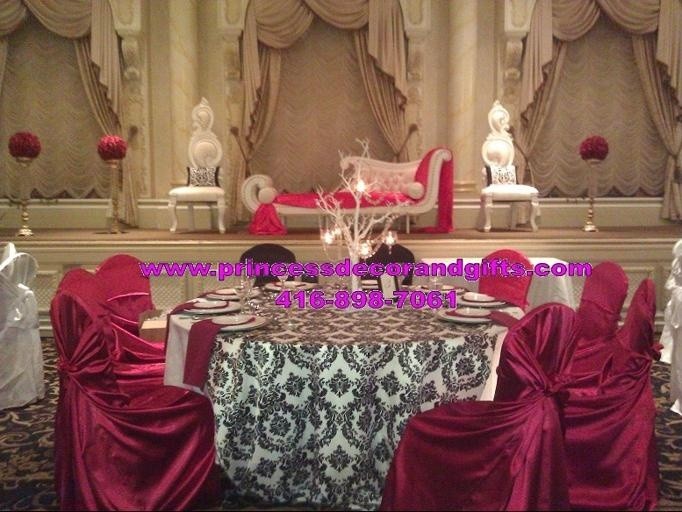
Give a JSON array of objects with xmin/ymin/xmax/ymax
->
[
  {"xmin": 183, "ymin": 300, "xmax": 242, "ymax": 314},
  {"xmin": 206, "ymin": 288, "xmax": 259, "ymax": 300},
  {"xmin": 454, "ymin": 292, "xmax": 506, "ymax": 306},
  {"xmin": 205, "ymin": 315, "xmax": 266, "ymax": 332},
  {"xmin": 437, "ymin": 308, "xmax": 495, "ymax": 323},
  {"xmin": 416, "ymin": 284, "xmax": 464, "ymax": 294},
  {"xmin": 265, "ymin": 280, "xmax": 314, "ymax": 291}
]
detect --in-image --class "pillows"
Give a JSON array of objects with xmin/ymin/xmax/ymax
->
[
  {"xmin": 184, "ymin": 165, "xmax": 223, "ymax": 187},
  {"xmin": 258, "ymin": 187, "xmax": 279, "ymax": 204},
  {"xmin": 483, "ymin": 164, "xmax": 520, "ymax": 186},
  {"xmin": 402, "ymin": 180, "xmax": 426, "ymax": 200}
]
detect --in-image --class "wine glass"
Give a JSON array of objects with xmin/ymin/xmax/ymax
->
[{"xmin": 233, "ymin": 269, "xmax": 256, "ymax": 313}]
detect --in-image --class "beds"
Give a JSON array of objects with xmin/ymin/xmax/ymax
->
[{"xmin": 240, "ymin": 148, "xmax": 453, "ymax": 235}]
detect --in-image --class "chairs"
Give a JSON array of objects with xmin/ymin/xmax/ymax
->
[
  {"xmin": 167, "ymin": 96, "xmax": 227, "ymax": 235},
  {"xmin": 0, "ymin": 237, "xmax": 682, "ymax": 512},
  {"xmin": 478, "ymin": 98, "xmax": 542, "ymax": 233}
]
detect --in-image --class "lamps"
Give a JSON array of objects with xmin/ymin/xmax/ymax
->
[{"xmin": 312, "ymin": 136, "xmax": 413, "ymax": 315}]
